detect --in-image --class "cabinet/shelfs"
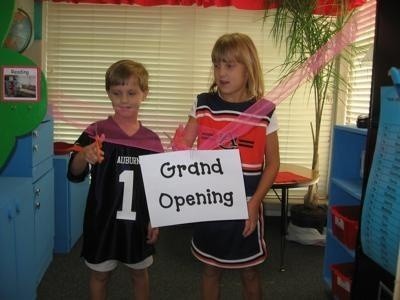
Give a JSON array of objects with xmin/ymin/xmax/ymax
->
[
  {"xmin": 0, "ymin": 103, "xmax": 90, "ymax": 300},
  {"xmin": 323, "ymin": 126, "xmax": 367, "ymax": 298}
]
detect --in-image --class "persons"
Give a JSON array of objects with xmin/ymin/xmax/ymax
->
[
  {"xmin": 67, "ymin": 60, "xmax": 165, "ymax": 300},
  {"xmin": 173, "ymin": 32, "xmax": 280, "ymax": 300}
]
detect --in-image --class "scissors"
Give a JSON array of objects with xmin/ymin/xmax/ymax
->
[{"xmin": 94, "ymin": 124, "xmax": 103, "ymax": 163}]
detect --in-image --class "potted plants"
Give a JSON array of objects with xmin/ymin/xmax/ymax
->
[{"xmin": 265, "ymin": 0, "xmax": 357, "ymax": 232}]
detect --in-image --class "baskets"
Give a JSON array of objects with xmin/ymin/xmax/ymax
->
[{"xmin": 329, "ymin": 262, "xmax": 354, "ymax": 300}]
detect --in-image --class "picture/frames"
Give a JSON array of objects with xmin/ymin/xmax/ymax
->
[{"xmin": 2, "ymin": 65, "xmax": 41, "ymax": 102}]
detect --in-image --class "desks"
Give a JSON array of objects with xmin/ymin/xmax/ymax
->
[{"xmin": 271, "ymin": 164, "xmax": 319, "ymax": 273}]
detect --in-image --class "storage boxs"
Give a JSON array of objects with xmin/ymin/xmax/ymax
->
[{"xmin": 329, "ymin": 264, "xmax": 353, "ymax": 296}]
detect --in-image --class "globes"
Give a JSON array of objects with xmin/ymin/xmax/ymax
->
[{"xmin": 4, "ymin": 8, "xmax": 33, "ymax": 52}]
